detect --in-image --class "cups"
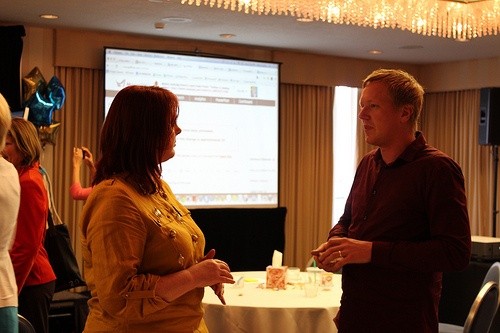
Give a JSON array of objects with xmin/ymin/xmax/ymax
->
[
  {"xmin": 286, "ymin": 267, "xmax": 300, "ymax": 285},
  {"xmin": 303, "ymin": 267, "xmax": 333, "ymax": 298}
]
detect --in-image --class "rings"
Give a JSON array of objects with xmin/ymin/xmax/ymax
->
[{"xmin": 339, "ymin": 251, "xmax": 341, "ymax": 256}]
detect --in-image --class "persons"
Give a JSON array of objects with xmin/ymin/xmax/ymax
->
[
  {"xmin": 71, "ymin": 146, "xmax": 98, "ymax": 200},
  {"xmin": 0, "ymin": 116, "xmax": 57, "ymax": 333},
  {"xmin": 309, "ymin": 69, "xmax": 471, "ymax": 333},
  {"xmin": 79, "ymin": 86, "xmax": 235, "ymax": 333},
  {"xmin": 0, "ymin": 92, "xmax": 20, "ymax": 333}
]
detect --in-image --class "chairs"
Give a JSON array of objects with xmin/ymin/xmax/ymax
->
[{"xmin": 438, "ymin": 261, "xmax": 500, "ymax": 333}]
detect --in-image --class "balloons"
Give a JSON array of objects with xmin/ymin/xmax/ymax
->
[{"xmin": 23, "ymin": 67, "xmax": 65, "ymax": 146}]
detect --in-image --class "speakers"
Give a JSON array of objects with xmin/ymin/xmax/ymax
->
[
  {"xmin": 479, "ymin": 87, "xmax": 500, "ymax": 145},
  {"xmin": 0, "ymin": 24, "xmax": 26, "ymax": 112}
]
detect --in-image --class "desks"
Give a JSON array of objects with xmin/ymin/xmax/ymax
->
[{"xmin": 200, "ymin": 269, "xmax": 342, "ymax": 333}]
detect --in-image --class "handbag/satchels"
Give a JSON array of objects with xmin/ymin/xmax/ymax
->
[{"xmin": 43, "ymin": 224, "xmax": 87, "ymax": 293}]
